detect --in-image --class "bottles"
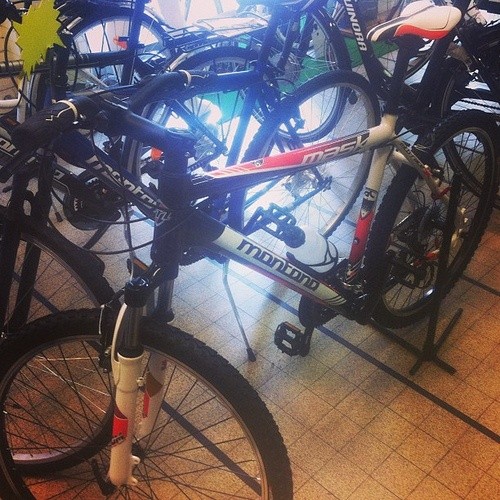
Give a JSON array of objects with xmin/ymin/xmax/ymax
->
[{"xmin": 283, "ymin": 225, "xmax": 339, "ymax": 277}]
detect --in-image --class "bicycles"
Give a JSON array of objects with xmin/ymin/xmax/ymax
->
[
  {"xmin": 236, "ymin": 1, "xmax": 448, "ymax": 145},
  {"xmin": 0, "ymin": 0, "xmax": 385, "ymax": 477},
  {"xmin": 1, "ymin": 1, "xmax": 500, "ymax": 500}
]
